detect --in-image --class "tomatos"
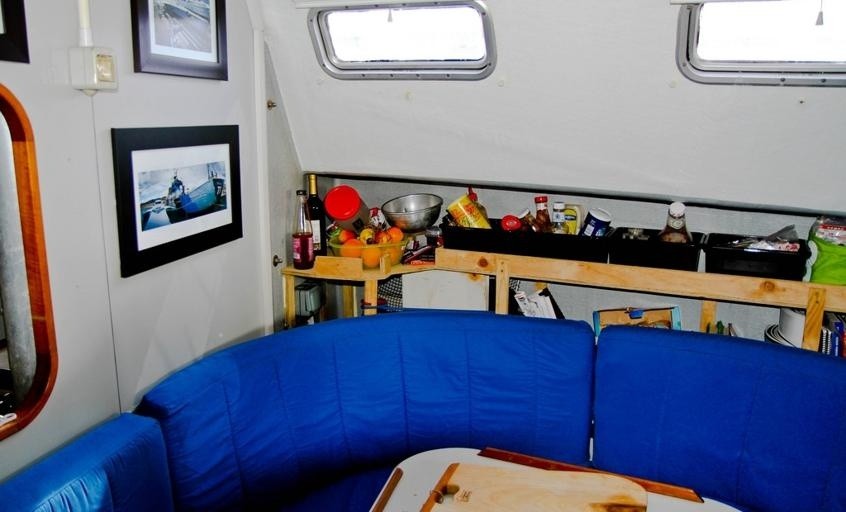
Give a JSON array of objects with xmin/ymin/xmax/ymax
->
[{"xmin": 501, "ymin": 214, "xmax": 522, "ymax": 233}]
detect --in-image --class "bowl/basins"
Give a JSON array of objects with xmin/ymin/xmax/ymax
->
[
  {"xmin": 329, "ymin": 240, "xmax": 408, "ymax": 271},
  {"xmin": 381, "ymin": 194, "xmax": 443, "ymax": 233}
]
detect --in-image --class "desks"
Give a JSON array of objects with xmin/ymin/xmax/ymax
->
[
  {"xmin": 365, "ymin": 443, "xmax": 740, "ymax": 512},
  {"xmin": 279, "ymin": 244, "xmax": 846, "ymax": 353}
]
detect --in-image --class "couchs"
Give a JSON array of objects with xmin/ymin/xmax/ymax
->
[
  {"xmin": 594, "ymin": 323, "xmax": 846, "ymax": 511},
  {"xmin": 129, "ymin": 311, "xmax": 594, "ymax": 511},
  {"xmin": 1, "ymin": 411, "xmax": 176, "ymax": 511}
]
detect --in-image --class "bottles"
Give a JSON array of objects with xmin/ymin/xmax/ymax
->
[
  {"xmin": 517, "ymin": 197, "xmax": 569, "ymax": 234},
  {"xmin": 654, "ymin": 201, "xmax": 694, "ymax": 244},
  {"xmin": 443, "ymin": 187, "xmax": 493, "ymax": 230},
  {"xmin": 323, "ymin": 185, "xmax": 371, "ymax": 234},
  {"xmin": 292, "ymin": 174, "xmax": 326, "ymax": 270}
]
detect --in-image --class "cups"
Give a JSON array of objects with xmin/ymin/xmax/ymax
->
[{"xmin": 577, "ymin": 207, "xmax": 612, "ymax": 237}]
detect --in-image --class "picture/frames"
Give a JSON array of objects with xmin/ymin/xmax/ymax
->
[
  {"xmin": 0, "ymin": 0, "xmax": 30, "ymax": 63},
  {"xmin": 111, "ymin": 124, "xmax": 243, "ymax": 278},
  {"xmin": 129, "ymin": 1, "xmax": 229, "ymax": 81}
]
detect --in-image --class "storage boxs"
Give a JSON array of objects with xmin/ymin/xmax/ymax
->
[
  {"xmin": 608, "ymin": 227, "xmax": 705, "ymax": 272},
  {"xmin": 703, "ymin": 233, "xmax": 812, "ymax": 281},
  {"xmin": 594, "ymin": 304, "xmax": 681, "ymax": 344},
  {"xmin": 441, "ymin": 218, "xmax": 608, "ymax": 264},
  {"xmin": 401, "ymin": 269, "xmax": 490, "ymax": 311}
]
